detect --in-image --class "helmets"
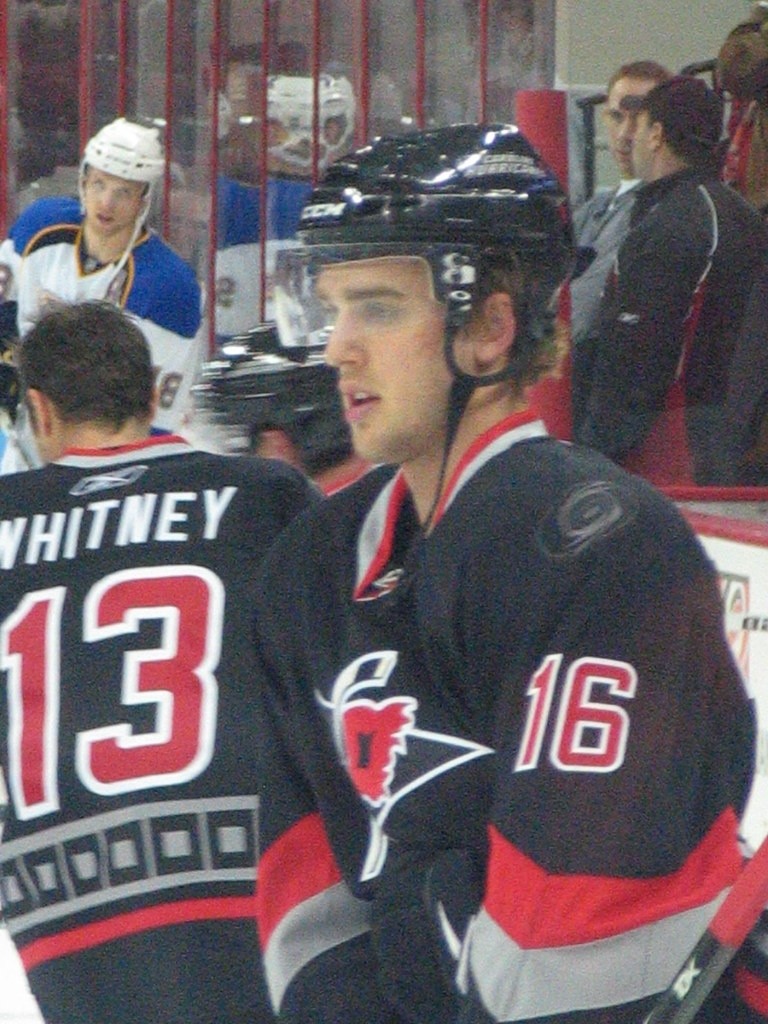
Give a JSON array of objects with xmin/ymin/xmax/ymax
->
[
  {"xmin": 265, "ymin": 74, "xmax": 354, "ymax": 168},
  {"xmin": 79, "ymin": 117, "xmax": 165, "ymax": 201},
  {"xmin": 185, "ymin": 320, "xmax": 354, "ymax": 456},
  {"xmin": 297, "ymin": 121, "xmax": 577, "ymax": 307}
]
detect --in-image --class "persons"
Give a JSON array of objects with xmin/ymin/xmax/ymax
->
[
  {"xmin": 190, "ymin": 309, "xmax": 368, "ymax": 497},
  {"xmin": 715, "ymin": 1, "xmax": 768, "ymax": 217},
  {"xmin": 578, "ymin": 79, "xmax": 768, "ymax": 488},
  {"xmin": 251, "ymin": 121, "xmax": 768, "ymax": 1023},
  {"xmin": 0, "ymin": 116, "xmax": 201, "ymax": 476},
  {"xmin": 1, "ymin": 302, "xmax": 330, "ymax": 1023},
  {"xmin": 569, "ymin": 64, "xmax": 676, "ymax": 345},
  {"xmin": 163, "ymin": 40, "xmax": 363, "ymax": 341}
]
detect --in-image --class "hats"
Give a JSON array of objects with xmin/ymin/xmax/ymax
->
[{"xmin": 620, "ymin": 75, "xmax": 722, "ymax": 151}]
